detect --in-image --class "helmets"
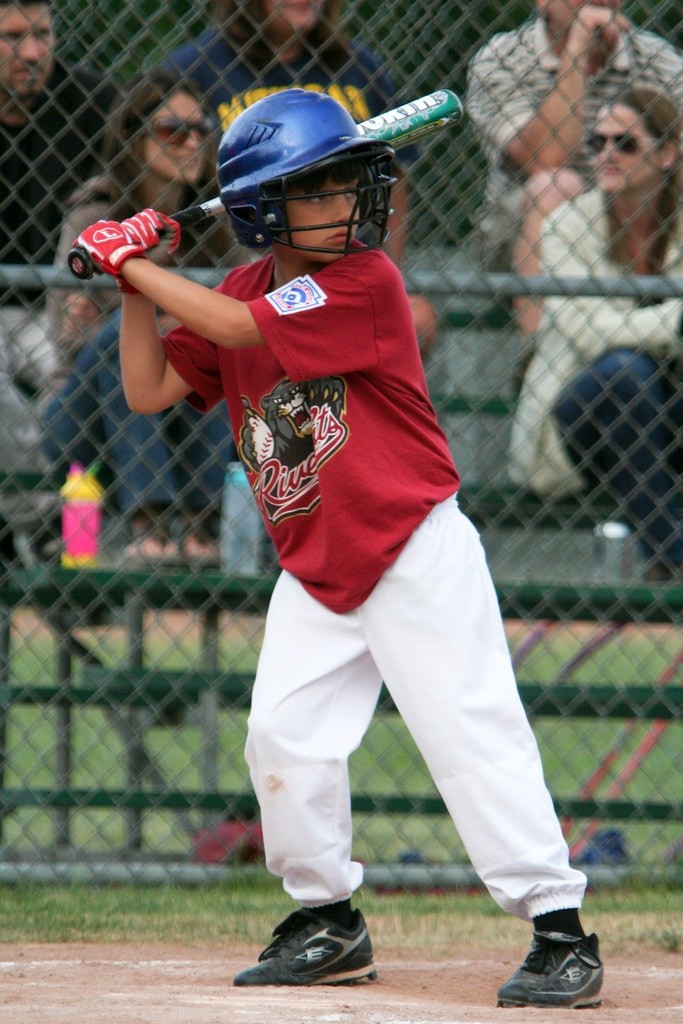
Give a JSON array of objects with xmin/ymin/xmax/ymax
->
[{"xmin": 215, "ymin": 88, "xmax": 394, "ymax": 248}]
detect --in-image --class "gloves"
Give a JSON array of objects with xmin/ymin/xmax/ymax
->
[
  {"xmin": 72, "ymin": 220, "xmax": 148, "ymax": 279},
  {"xmin": 114, "ymin": 207, "xmax": 180, "ymax": 297}
]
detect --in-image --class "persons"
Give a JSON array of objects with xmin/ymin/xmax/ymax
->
[
  {"xmin": 73, "ymin": 87, "xmax": 607, "ymax": 1013},
  {"xmin": 0, "ymin": 0, "xmax": 683, "ymax": 581}
]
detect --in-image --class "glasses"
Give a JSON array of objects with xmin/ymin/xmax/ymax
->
[
  {"xmin": 585, "ymin": 128, "xmax": 667, "ymax": 154},
  {"xmin": 137, "ymin": 115, "xmax": 217, "ymax": 146}
]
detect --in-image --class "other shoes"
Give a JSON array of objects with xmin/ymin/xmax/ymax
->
[
  {"xmin": 512, "ymin": 352, "xmax": 536, "ymax": 393},
  {"xmin": 639, "ymin": 552, "xmax": 683, "ymax": 584}
]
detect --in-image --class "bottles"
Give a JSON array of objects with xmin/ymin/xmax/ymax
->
[{"xmin": 61, "ymin": 464, "xmax": 103, "ymax": 570}]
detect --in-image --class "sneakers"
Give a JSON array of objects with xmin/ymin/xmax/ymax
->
[
  {"xmin": 497, "ymin": 931, "xmax": 604, "ymax": 1009},
  {"xmin": 233, "ymin": 908, "xmax": 377, "ymax": 987}
]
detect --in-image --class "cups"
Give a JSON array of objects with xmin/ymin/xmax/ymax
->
[
  {"xmin": 220, "ymin": 462, "xmax": 263, "ymax": 577},
  {"xmin": 593, "ymin": 522, "xmax": 633, "ymax": 585}
]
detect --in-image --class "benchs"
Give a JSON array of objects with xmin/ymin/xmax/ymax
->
[{"xmin": 0, "ymin": 243, "xmax": 683, "ymax": 583}]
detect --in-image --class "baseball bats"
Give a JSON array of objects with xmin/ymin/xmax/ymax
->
[{"xmin": 67, "ymin": 87, "xmax": 464, "ymax": 279}]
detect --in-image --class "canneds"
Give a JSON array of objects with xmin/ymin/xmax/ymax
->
[{"xmin": 593, "ymin": 521, "xmax": 631, "ymax": 582}]
